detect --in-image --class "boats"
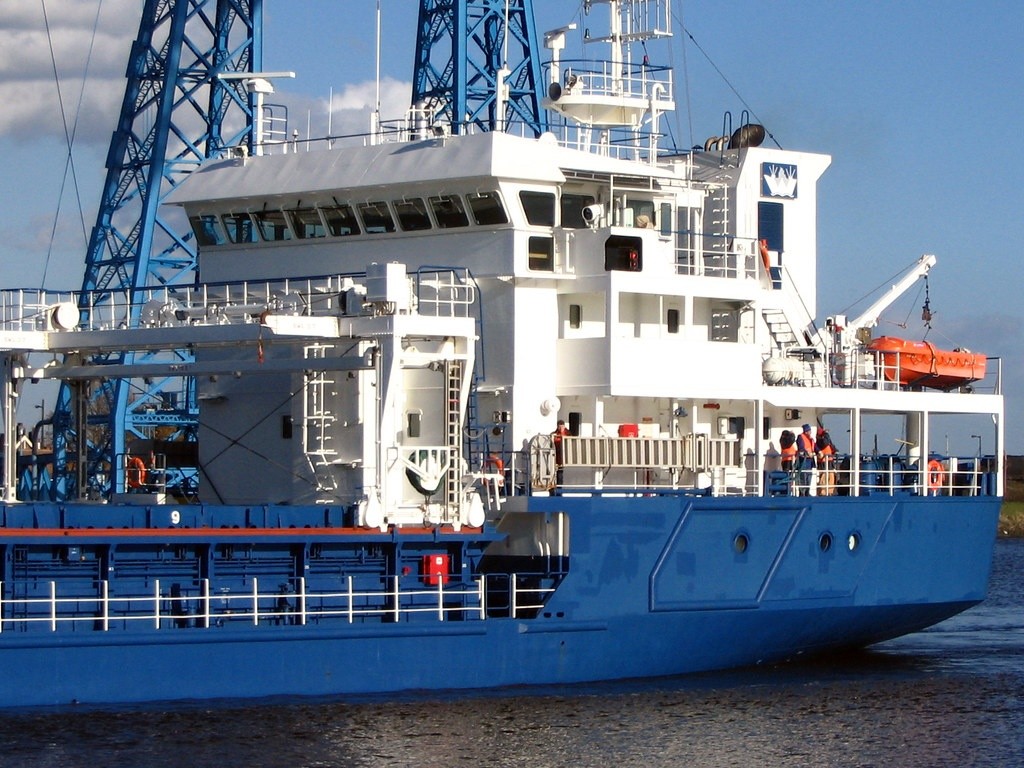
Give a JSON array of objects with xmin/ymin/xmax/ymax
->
[{"xmin": 868, "ymin": 335, "xmax": 987, "ymax": 389}]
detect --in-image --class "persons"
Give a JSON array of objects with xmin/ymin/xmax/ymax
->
[
  {"xmin": 816, "ymin": 430, "xmax": 839, "ymax": 469},
  {"xmin": 781, "ymin": 430, "xmax": 796, "ymax": 471},
  {"xmin": 554, "ymin": 421, "xmax": 572, "ymax": 464},
  {"xmin": 796, "ymin": 424, "xmax": 822, "ymax": 497}
]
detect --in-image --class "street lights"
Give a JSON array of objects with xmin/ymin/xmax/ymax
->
[{"xmin": 970, "ymin": 435, "xmax": 981, "ymax": 459}]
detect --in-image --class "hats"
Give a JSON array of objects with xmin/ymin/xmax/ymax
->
[{"xmin": 802, "ymin": 424, "xmax": 811, "ymax": 432}]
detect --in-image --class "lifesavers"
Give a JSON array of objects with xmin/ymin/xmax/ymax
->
[
  {"xmin": 928, "ymin": 460, "xmax": 943, "ymax": 491},
  {"xmin": 127, "ymin": 457, "xmax": 145, "ymax": 488},
  {"xmin": 761, "ymin": 247, "xmax": 770, "ymax": 275},
  {"xmin": 481, "ymin": 455, "xmax": 503, "ymax": 485}
]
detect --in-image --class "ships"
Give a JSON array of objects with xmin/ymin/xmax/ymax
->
[{"xmin": 0, "ymin": 0, "xmax": 1007, "ymax": 717}]
